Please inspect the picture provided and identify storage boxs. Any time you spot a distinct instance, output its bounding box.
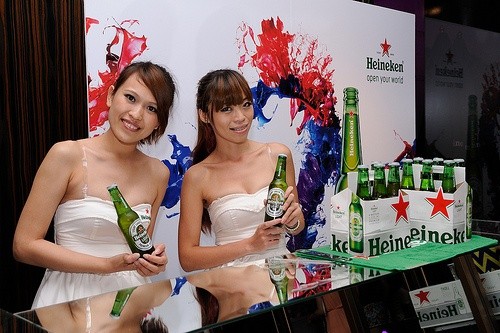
[330,166,473,258]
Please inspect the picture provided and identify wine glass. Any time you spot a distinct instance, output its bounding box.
[109,286,139,320]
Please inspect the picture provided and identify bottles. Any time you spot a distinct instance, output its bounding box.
[441,160,456,193]
[465,94,482,219]
[419,159,435,191]
[334,86,363,195]
[413,157,424,164]
[386,162,400,198]
[432,158,444,166]
[400,158,415,190]
[348,192,364,254]
[371,163,387,200]
[466,185,472,239]
[264,254,288,305]
[264,154,287,242]
[371,161,381,170]
[106,183,155,258]
[355,165,372,200]
[349,265,364,285]
[453,159,465,167]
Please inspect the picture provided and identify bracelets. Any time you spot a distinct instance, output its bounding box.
[283,220,300,232]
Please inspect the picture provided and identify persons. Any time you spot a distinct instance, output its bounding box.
[35,279,172,333]
[12,63,176,311]
[186,253,297,329]
[178,71,305,272]
